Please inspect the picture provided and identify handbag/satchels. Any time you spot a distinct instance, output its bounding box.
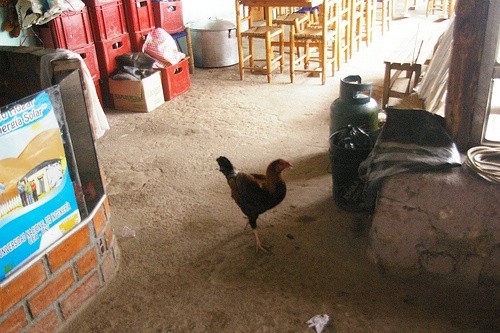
[142,27,186,69]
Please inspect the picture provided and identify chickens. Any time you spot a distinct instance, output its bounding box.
[215,155,294,255]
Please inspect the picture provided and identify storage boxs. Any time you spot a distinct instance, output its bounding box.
[35,0,191,112]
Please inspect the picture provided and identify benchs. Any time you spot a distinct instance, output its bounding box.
[381,35,431,110]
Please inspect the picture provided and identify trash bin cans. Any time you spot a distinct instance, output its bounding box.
[324,125,373,216]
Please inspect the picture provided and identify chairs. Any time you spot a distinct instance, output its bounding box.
[235,0,455,85]
